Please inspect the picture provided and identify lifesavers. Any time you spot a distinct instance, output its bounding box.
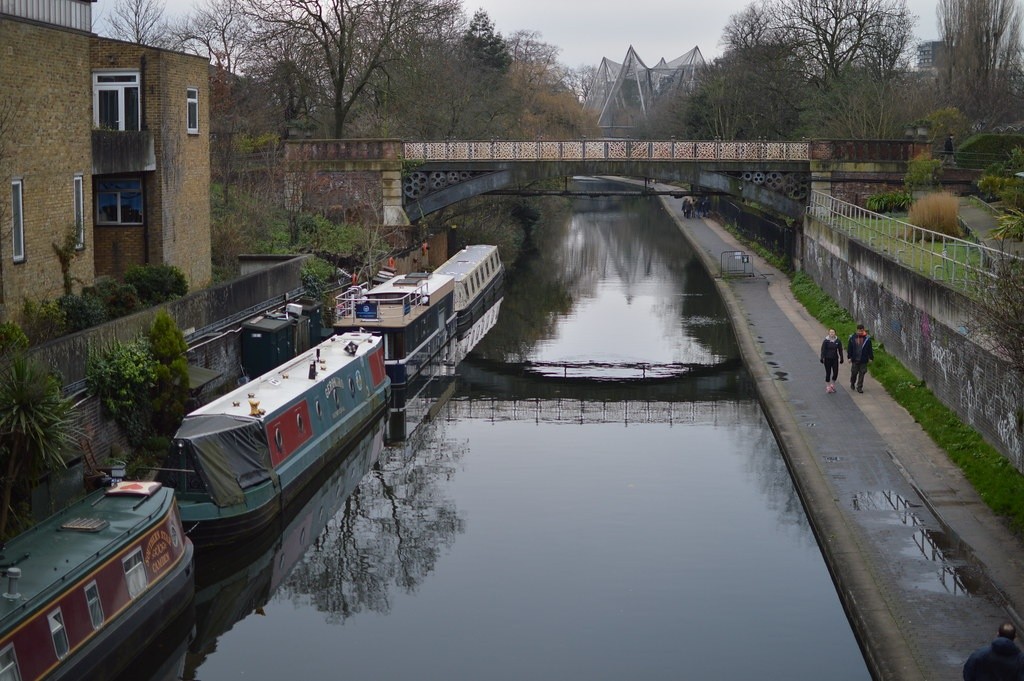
[422,242,428,254]
[352,272,358,286]
[388,256,395,270]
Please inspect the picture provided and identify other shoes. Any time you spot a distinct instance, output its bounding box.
[851,383,855,389]
[857,386,863,393]
[826,383,836,392]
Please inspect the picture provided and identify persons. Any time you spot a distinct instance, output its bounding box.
[681,197,710,219]
[847,325,874,393]
[941,134,954,167]
[820,328,843,393]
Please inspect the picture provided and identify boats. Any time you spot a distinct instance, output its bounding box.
[389,336,458,464]
[0,481,194,681]
[332,272,457,407]
[154,327,392,533]
[44,535,197,681]
[431,245,504,316]
[188,408,392,614]
[457,274,504,361]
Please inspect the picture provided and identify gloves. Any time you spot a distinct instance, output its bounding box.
[840,360,843,364]
[820,359,823,363]
